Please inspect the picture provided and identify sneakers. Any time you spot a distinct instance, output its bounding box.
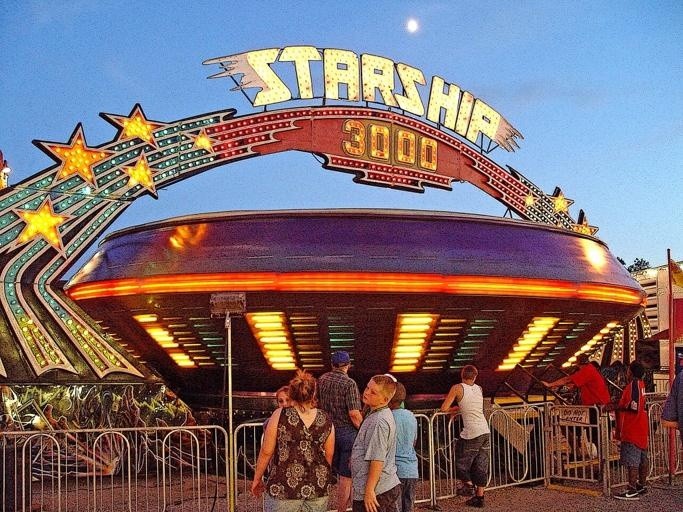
[614,482,649,500]
[456,483,485,507]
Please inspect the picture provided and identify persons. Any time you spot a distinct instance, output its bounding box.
[388,382,419,512]
[661,369,683,445]
[540,353,651,501]
[318,351,364,512]
[263,386,291,433]
[251,369,335,512]
[349,375,401,512]
[440,365,491,508]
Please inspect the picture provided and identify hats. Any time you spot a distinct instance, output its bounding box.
[571,353,588,364]
[331,351,350,363]
